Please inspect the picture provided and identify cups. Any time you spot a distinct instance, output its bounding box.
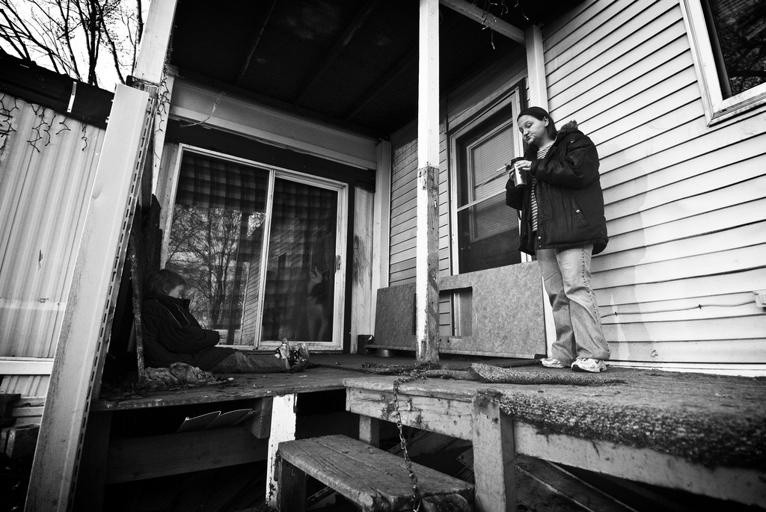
[510,156,529,188]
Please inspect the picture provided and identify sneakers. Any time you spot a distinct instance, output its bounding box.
[571,359,607,373]
[274,338,310,371]
[539,358,570,369]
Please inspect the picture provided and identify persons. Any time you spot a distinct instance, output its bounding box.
[142,269,311,373]
[505,107,612,372]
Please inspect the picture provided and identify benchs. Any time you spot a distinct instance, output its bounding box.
[271,432,475,512]
